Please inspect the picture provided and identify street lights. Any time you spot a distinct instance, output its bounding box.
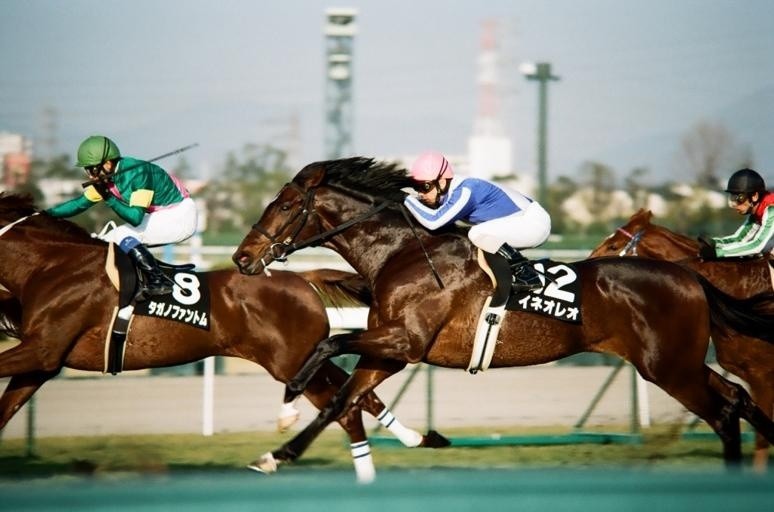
[319,3,359,159]
[526,57,560,235]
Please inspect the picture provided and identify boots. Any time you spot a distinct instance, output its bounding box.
[128,244,173,295]
[494,242,542,291]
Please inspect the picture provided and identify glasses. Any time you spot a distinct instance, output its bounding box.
[413,181,435,193]
[85,167,100,176]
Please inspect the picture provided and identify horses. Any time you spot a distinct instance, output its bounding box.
[0,191,452,484]
[230,156,774,476]
[588,208,774,473]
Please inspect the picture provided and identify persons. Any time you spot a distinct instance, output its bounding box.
[47,135,199,304]
[403,149,552,293]
[698,169,774,261]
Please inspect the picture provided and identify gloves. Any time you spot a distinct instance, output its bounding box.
[698,246,716,262]
[93,177,111,200]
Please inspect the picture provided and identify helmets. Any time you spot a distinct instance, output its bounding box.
[409,150,454,182]
[725,169,765,193]
[75,135,120,167]
